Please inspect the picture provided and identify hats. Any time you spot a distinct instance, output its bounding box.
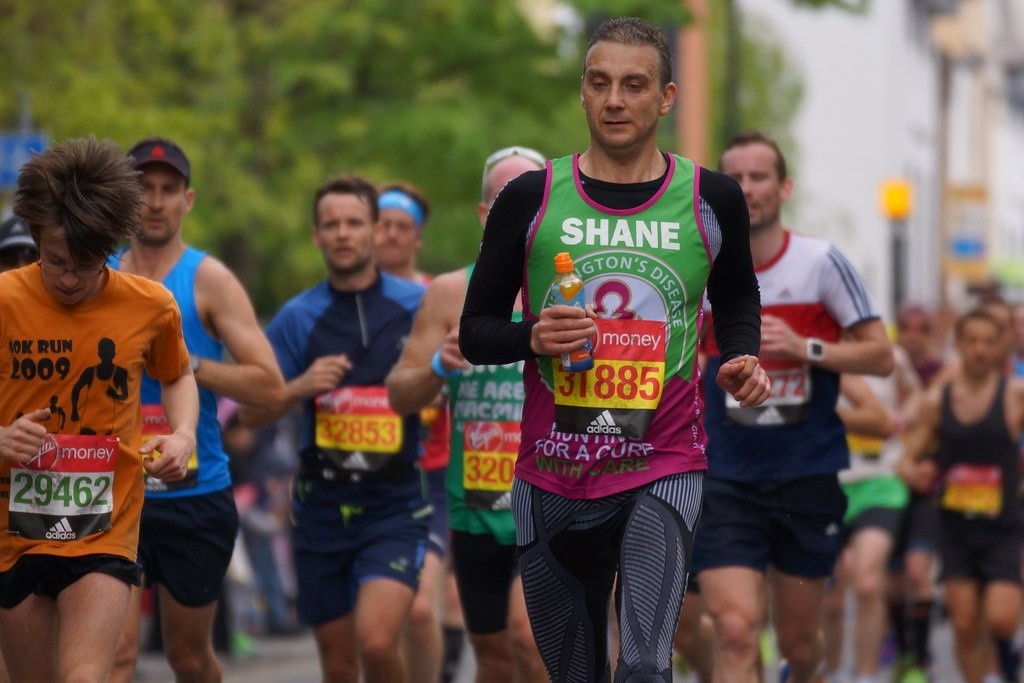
[125,137,191,186]
[0,217,37,249]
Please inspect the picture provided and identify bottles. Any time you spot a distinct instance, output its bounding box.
[549,252,594,372]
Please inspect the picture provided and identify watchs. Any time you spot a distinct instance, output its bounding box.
[189,355,200,372]
[803,338,825,370]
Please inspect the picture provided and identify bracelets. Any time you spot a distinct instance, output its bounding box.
[431,351,461,379]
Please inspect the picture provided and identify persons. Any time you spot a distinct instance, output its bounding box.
[0,139,285,683]
[218,146,559,683]
[461,15,771,683]
[677,133,1024,683]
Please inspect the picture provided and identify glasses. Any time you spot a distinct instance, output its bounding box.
[36,252,109,278]
